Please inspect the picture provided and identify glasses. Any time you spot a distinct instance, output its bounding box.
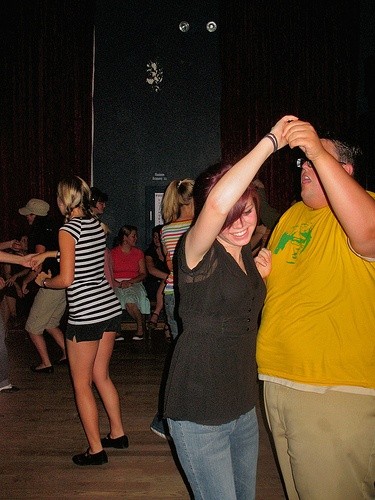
[296,157,347,169]
[154,234,160,239]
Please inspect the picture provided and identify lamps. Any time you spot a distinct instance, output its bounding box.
[145,56,164,95]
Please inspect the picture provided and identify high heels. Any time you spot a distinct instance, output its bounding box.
[148,312,159,327]
[162,327,173,345]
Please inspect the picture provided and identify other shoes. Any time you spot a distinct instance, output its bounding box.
[72,447,108,467]
[100,432,129,449]
[150,415,171,440]
[54,358,69,366]
[115,334,124,341]
[132,332,144,340]
[0,383,20,392]
[30,364,54,374]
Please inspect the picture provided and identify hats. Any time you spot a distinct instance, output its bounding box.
[18,199,50,216]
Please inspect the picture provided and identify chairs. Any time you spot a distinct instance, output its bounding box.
[119,302,168,341]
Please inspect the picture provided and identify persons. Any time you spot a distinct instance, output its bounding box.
[250,178,303,259]
[0,173,197,466]
[255,121,375,500]
[161,114,299,500]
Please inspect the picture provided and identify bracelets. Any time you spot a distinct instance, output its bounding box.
[263,133,279,154]
[258,183,264,188]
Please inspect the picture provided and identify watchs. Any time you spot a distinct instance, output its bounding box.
[43,278,51,289]
[119,282,122,288]
